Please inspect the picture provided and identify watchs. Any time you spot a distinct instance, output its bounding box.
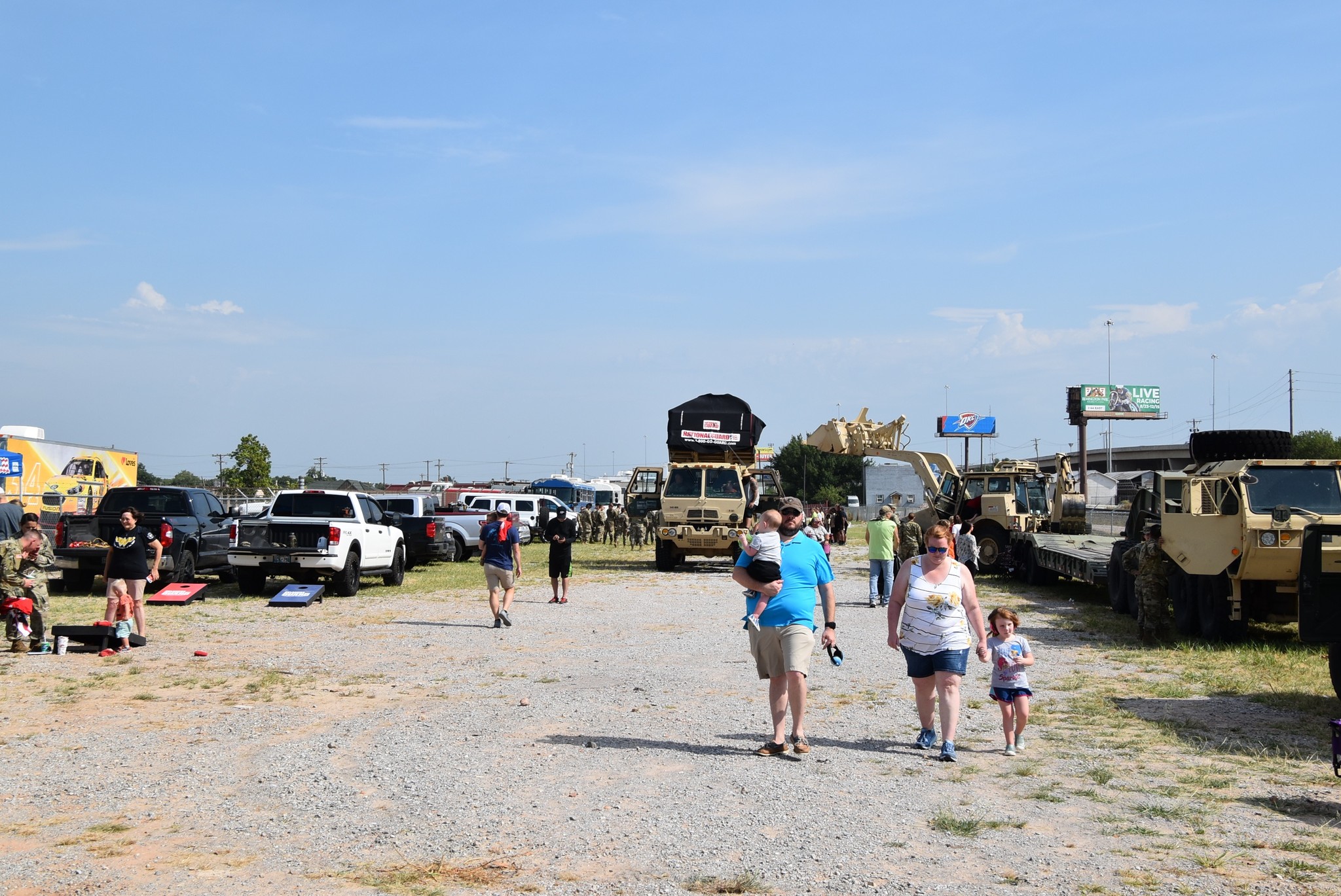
[825,621,836,629]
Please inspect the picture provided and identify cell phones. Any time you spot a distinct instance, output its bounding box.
[558,538,563,540]
[28,547,40,556]
[977,546,981,549]
[146,574,153,583]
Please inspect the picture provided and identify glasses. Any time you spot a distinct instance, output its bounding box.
[119,518,131,522]
[782,508,800,516]
[928,546,948,554]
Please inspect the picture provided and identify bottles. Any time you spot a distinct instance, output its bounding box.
[288,533,296,548]
[317,536,327,550]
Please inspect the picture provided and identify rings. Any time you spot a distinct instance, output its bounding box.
[834,644,835,645]
[31,555,33,557]
[751,506,752,506]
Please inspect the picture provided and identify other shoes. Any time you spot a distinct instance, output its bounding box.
[479,558,484,566]
[115,645,132,653]
[583,539,654,551]
[1006,744,1016,756]
[1014,730,1026,750]
[742,589,756,599]
[869,601,888,607]
[748,614,760,632]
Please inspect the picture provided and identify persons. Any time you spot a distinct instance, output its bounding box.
[576,502,660,550]
[103,506,163,637]
[886,503,901,556]
[479,502,522,628]
[0,529,43,653]
[732,496,836,756]
[937,515,980,580]
[111,579,133,653]
[721,468,760,529]
[545,505,576,604]
[724,480,739,492]
[978,608,1034,756]
[886,525,988,762]
[800,503,849,546]
[5,513,56,649]
[865,505,900,608]
[0,486,27,542]
[479,513,498,566]
[899,512,923,564]
[739,510,783,633]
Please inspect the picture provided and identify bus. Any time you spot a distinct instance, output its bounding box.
[523,474,597,515]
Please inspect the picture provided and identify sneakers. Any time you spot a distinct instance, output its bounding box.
[939,740,958,762]
[790,733,810,754]
[499,610,511,626]
[756,741,788,756]
[548,597,559,603]
[494,620,501,628]
[30,639,52,651]
[10,640,30,652]
[915,727,936,750]
[559,598,568,604]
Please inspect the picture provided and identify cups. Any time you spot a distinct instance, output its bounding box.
[57,636,69,655]
[41,642,50,652]
[738,534,753,550]
[25,575,36,579]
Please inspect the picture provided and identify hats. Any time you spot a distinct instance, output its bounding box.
[741,469,752,477]
[887,503,897,508]
[778,497,803,512]
[497,502,511,514]
[879,506,893,514]
[557,507,566,519]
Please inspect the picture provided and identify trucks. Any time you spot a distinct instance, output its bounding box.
[623,393,790,569]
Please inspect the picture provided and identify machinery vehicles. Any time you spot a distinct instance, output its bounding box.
[801,408,1056,577]
[1018,427,1341,647]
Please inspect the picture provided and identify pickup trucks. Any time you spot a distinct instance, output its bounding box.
[366,489,582,570]
[228,488,407,597]
[50,484,240,592]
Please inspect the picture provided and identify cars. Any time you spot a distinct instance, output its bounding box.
[40,456,110,518]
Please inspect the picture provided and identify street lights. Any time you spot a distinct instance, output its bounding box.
[583,442,587,481]
[944,385,950,456]
[1211,354,1219,430]
[612,450,616,476]
[1104,319,1114,473]
[643,435,648,467]
